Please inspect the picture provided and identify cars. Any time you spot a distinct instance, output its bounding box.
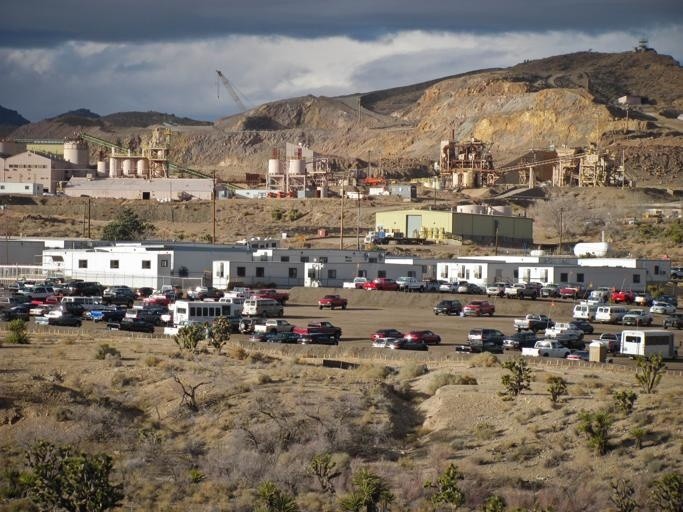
[435,300,462,316]
[370,328,441,351]
[1,277,178,333]
[590,286,653,305]
[661,314,683,328]
[503,332,538,350]
[298,334,338,345]
[671,268,683,279]
[601,332,620,350]
[650,303,677,314]
[427,280,454,291]
[546,319,593,350]
[652,294,677,305]
[458,281,470,292]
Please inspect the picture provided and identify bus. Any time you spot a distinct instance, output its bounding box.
[173,301,244,328]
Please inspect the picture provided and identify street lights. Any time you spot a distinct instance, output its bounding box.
[81,194,91,238]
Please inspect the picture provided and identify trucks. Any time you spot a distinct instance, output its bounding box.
[573,304,598,321]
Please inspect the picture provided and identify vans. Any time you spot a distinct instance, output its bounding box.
[243,299,284,317]
[596,306,628,324]
[468,329,510,346]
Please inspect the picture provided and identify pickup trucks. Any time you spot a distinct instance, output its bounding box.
[342,276,367,289]
[623,309,653,327]
[464,300,495,317]
[396,276,427,292]
[522,339,571,358]
[307,321,341,336]
[487,282,585,300]
[250,288,289,301]
[514,314,555,331]
[255,320,294,334]
[319,295,347,310]
[362,278,397,289]
